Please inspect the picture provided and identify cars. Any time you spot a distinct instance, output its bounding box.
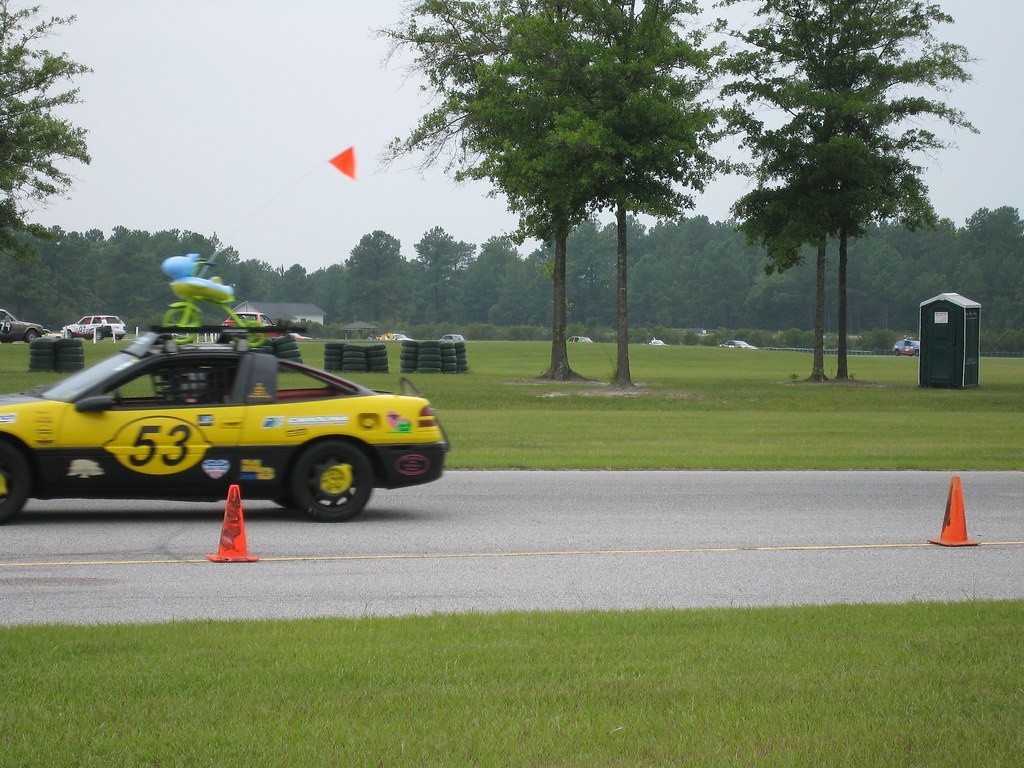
[718,340,758,349]
[0,309,45,344]
[566,336,593,343]
[61,315,128,340]
[649,338,665,345]
[892,339,919,357]
[439,334,465,341]
[0,325,450,523]
[42,329,62,340]
[223,312,415,341]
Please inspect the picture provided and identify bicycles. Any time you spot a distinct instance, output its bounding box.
[161,253,266,348]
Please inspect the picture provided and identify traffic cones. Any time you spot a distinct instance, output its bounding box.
[927,477,981,547]
[207,485,259,563]
